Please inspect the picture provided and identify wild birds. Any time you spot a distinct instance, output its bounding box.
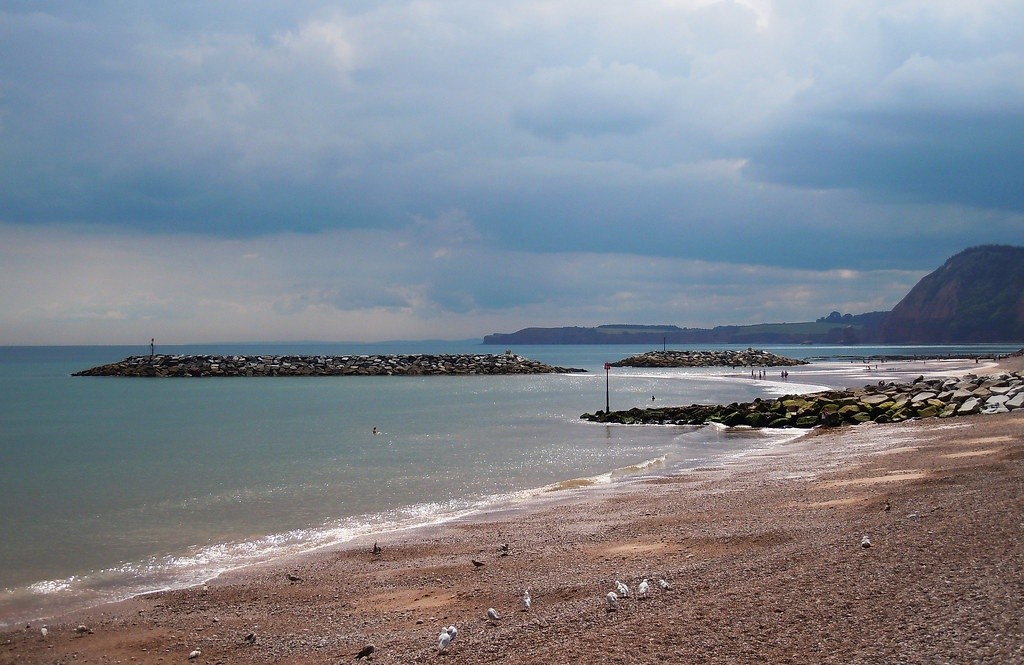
[614,580,629,599]
[189,647,201,659]
[40,625,48,639]
[500,544,510,555]
[245,630,257,645]
[437,625,458,654]
[657,580,673,591]
[471,560,485,571]
[638,579,650,599]
[860,535,871,549]
[487,608,499,625]
[606,592,618,612]
[884,503,891,512]
[202,583,209,595]
[73,625,90,637]
[353,644,375,662]
[373,543,381,554]
[522,592,531,612]
[286,574,302,586]
